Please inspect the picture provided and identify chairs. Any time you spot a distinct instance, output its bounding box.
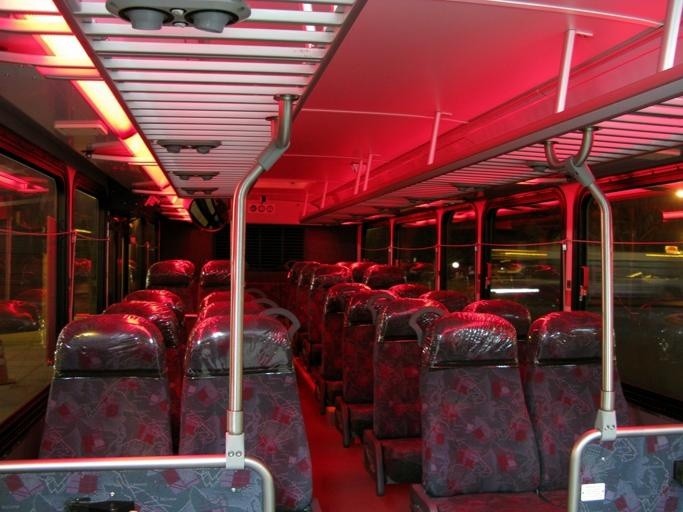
[0,249,682,512]
